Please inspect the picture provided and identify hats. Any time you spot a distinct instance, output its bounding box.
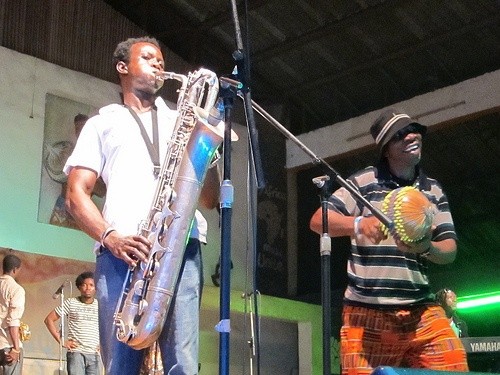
[370,109,428,161]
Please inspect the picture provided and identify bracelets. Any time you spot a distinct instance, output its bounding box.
[353,216,365,240]
[100,227,116,249]
[11,348,20,354]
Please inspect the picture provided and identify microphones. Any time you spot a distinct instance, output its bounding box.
[52,280,67,299]
[241,289,258,297]
[218,76,243,89]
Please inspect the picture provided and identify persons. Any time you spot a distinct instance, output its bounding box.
[0,255,30,375]
[44,271,105,375]
[307,109,471,373]
[64,37,222,374]
[50,112,106,229]
[434,287,469,339]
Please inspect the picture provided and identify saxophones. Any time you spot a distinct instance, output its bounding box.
[111,66,240,351]
